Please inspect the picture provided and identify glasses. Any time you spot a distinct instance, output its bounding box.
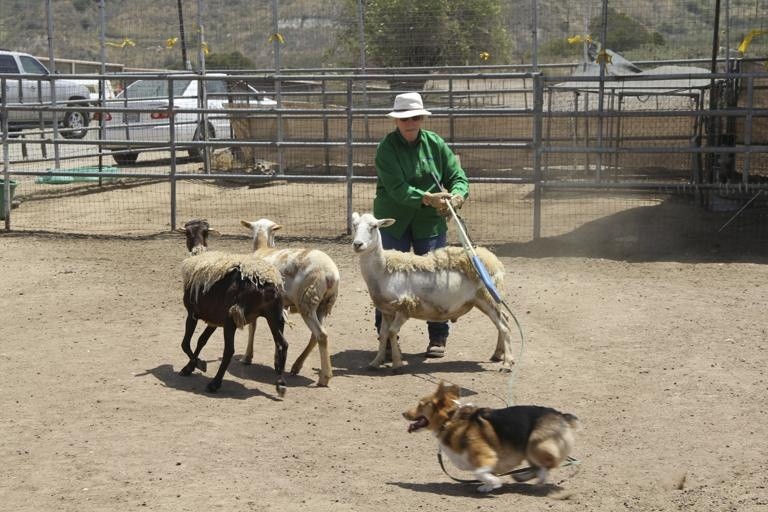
[399,116,421,121]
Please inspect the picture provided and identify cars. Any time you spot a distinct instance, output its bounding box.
[72,73,115,120]
[0,49,90,140]
[102,72,277,166]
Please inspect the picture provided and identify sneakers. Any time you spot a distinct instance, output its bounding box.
[379,336,402,361]
[426,337,445,357]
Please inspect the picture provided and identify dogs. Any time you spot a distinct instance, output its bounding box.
[401,381,584,493]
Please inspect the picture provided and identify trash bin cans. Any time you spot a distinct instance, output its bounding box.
[0,179,18,220]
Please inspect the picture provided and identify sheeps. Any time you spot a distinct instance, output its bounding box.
[238,217,340,387]
[175,218,290,398]
[351,211,517,374]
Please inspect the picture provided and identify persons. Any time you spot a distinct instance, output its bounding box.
[368,91,470,359]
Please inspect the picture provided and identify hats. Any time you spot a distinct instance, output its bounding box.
[384,92,432,118]
